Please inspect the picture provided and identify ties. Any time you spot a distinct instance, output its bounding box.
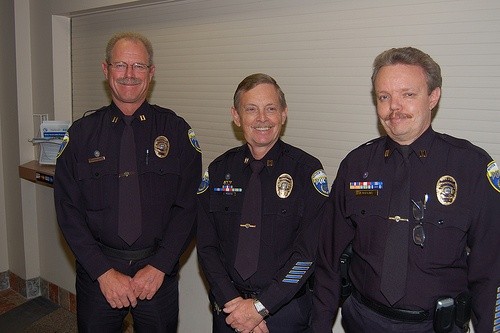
[234,160,264,281]
[380,145,413,305]
[118,116,142,246]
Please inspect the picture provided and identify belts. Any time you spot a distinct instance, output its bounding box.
[95,242,160,259]
[239,282,309,299]
[351,285,435,322]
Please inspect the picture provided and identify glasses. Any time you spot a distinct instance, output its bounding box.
[411,200,425,247]
[107,62,152,72]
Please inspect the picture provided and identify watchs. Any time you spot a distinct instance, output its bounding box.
[253,297,268,318]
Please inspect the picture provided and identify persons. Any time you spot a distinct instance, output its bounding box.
[330,47,500,333]
[53,32,202,333]
[194,74,339,333]
